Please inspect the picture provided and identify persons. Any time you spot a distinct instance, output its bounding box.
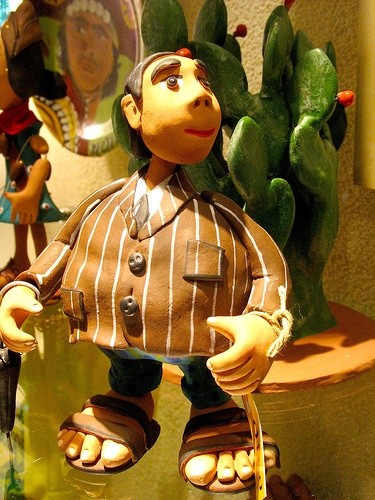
[0,49,294,493]
